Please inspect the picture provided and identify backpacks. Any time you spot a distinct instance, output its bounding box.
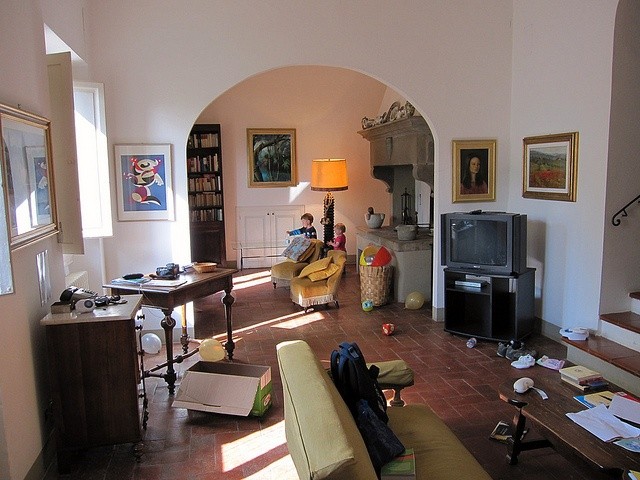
[331,342,389,425]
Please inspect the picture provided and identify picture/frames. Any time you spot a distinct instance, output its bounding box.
[113,143,175,222]
[523,132,579,202]
[0,153,15,295]
[453,140,497,201]
[247,128,297,186]
[0,103,59,252]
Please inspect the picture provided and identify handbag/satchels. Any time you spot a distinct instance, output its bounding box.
[356,399,405,471]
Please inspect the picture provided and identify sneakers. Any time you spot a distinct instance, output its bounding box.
[505,342,539,362]
[497,339,519,357]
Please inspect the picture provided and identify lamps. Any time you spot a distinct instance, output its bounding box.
[311,159,348,247]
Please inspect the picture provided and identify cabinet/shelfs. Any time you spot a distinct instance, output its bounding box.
[443,268,536,344]
[186,124,227,267]
[40,295,148,463]
[236,206,305,268]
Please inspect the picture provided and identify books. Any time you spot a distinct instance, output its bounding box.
[187,153,220,172]
[381,447,415,480]
[193,208,223,221]
[559,365,603,390]
[584,391,618,407]
[573,395,595,409]
[187,133,218,148]
[191,192,223,206]
[189,173,222,191]
[490,419,529,445]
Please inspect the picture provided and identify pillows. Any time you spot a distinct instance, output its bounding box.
[298,255,332,278]
[282,236,310,261]
[298,241,316,261]
[285,248,315,263]
[308,263,339,282]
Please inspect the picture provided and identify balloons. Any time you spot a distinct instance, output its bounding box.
[141,333,163,353]
[199,338,227,362]
[404,292,423,311]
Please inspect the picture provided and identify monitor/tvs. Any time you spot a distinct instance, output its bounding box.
[440,212,528,275]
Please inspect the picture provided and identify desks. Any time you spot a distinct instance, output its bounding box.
[497,373,640,480]
[356,225,433,303]
[103,267,238,394]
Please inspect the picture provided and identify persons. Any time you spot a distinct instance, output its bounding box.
[287,213,317,240]
[461,154,489,193]
[327,223,347,274]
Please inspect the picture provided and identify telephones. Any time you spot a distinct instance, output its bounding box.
[57,286,98,307]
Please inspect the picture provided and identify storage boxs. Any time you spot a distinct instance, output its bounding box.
[172,361,273,419]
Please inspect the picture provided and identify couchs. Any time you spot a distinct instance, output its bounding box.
[270,240,322,289]
[275,340,492,480]
[291,250,346,313]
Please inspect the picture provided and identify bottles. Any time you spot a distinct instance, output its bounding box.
[284,232,290,248]
